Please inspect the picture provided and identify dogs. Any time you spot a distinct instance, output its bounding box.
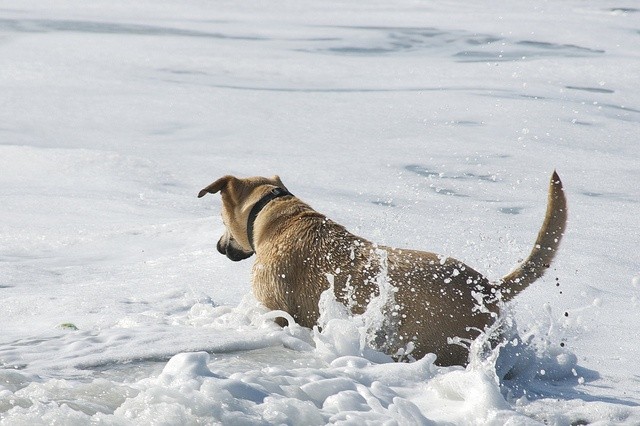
[198,168,567,367]
